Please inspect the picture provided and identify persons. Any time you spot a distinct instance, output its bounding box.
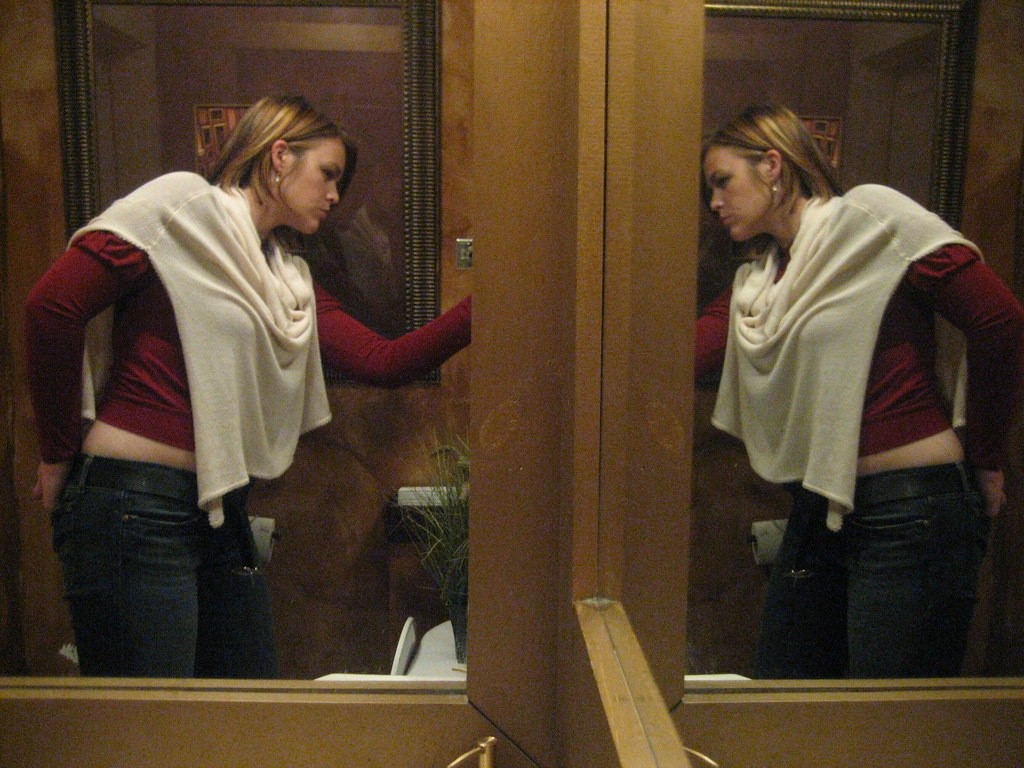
[695,99,1024,677]
[19,91,473,677]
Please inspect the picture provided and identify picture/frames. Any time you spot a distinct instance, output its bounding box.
[52,0,444,389]
[717,0,979,315]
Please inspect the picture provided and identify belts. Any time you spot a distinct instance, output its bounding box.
[72,460,197,504]
[855,467,974,508]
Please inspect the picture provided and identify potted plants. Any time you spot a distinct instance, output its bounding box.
[386,418,469,664]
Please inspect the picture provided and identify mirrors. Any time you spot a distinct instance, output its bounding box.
[0,0,657,768]
[603,0,1024,768]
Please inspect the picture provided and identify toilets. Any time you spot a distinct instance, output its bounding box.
[391,617,466,679]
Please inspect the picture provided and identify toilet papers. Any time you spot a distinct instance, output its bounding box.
[248,516,275,564]
[752,519,788,565]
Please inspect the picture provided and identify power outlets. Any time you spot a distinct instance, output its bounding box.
[456,239,473,269]
